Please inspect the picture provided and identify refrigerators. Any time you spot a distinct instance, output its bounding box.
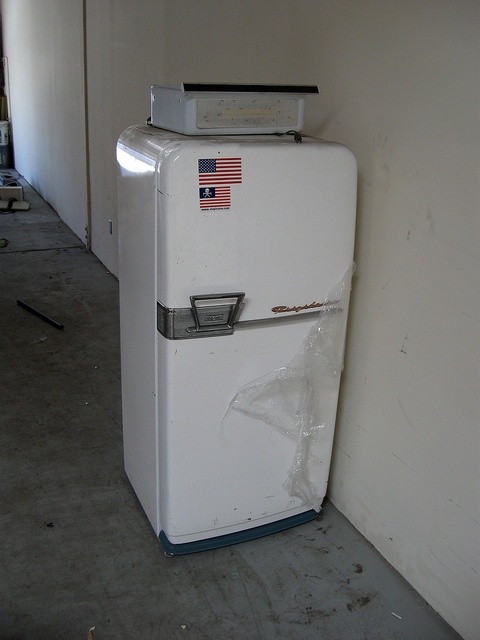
[116,126,360,557]
